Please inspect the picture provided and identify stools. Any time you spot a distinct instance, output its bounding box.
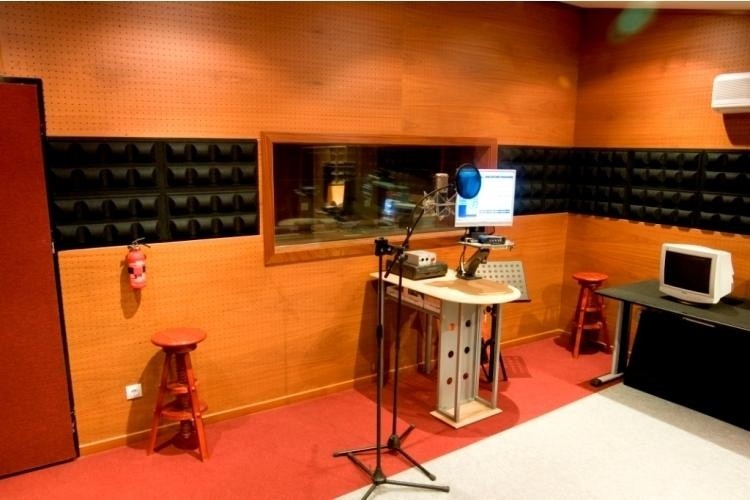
[572,272,613,359]
[147,327,209,461]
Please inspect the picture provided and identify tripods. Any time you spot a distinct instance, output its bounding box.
[332,245,436,482]
[347,239,449,500]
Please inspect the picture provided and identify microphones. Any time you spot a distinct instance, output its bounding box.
[433,172,448,217]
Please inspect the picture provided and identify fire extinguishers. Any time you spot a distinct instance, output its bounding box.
[126,238,150,289]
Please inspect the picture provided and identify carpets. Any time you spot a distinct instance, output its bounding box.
[0,335,626,500]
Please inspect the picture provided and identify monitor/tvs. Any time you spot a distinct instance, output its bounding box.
[658,243,734,305]
[454,168,517,242]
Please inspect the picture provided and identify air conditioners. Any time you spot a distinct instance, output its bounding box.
[707,72,750,112]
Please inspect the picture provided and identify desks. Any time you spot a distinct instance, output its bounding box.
[588,280,750,398]
[369,272,522,429]
